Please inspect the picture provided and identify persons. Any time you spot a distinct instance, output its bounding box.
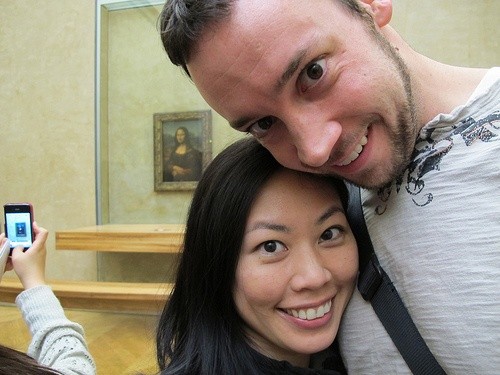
[156,0,500,375]
[155,136,359,375]
[0,221,96,375]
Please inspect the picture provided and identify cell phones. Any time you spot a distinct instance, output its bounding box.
[3,204,35,255]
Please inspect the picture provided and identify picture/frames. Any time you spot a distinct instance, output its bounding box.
[153,109,213,191]
